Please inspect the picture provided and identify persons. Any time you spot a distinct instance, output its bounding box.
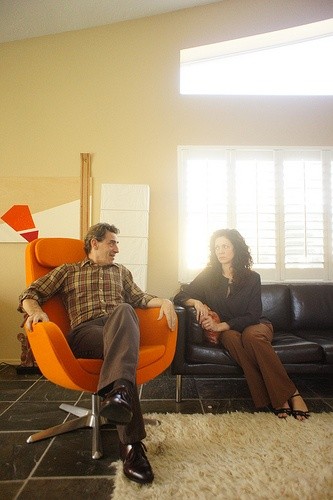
[173,229,311,421]
[17,222,176,486]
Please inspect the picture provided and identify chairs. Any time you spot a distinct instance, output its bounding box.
[25,238,178,459]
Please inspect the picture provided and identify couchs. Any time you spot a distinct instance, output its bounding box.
[173,281,333,403]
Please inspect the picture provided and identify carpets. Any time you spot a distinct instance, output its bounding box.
[111,411,333,499]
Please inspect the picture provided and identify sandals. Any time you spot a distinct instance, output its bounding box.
[290,393,310,420]
[273,403,291,418]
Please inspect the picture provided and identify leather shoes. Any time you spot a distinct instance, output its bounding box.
[118,439,155,483]
[100,385,134,422]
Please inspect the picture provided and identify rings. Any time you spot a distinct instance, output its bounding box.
[200,311,205,316]
